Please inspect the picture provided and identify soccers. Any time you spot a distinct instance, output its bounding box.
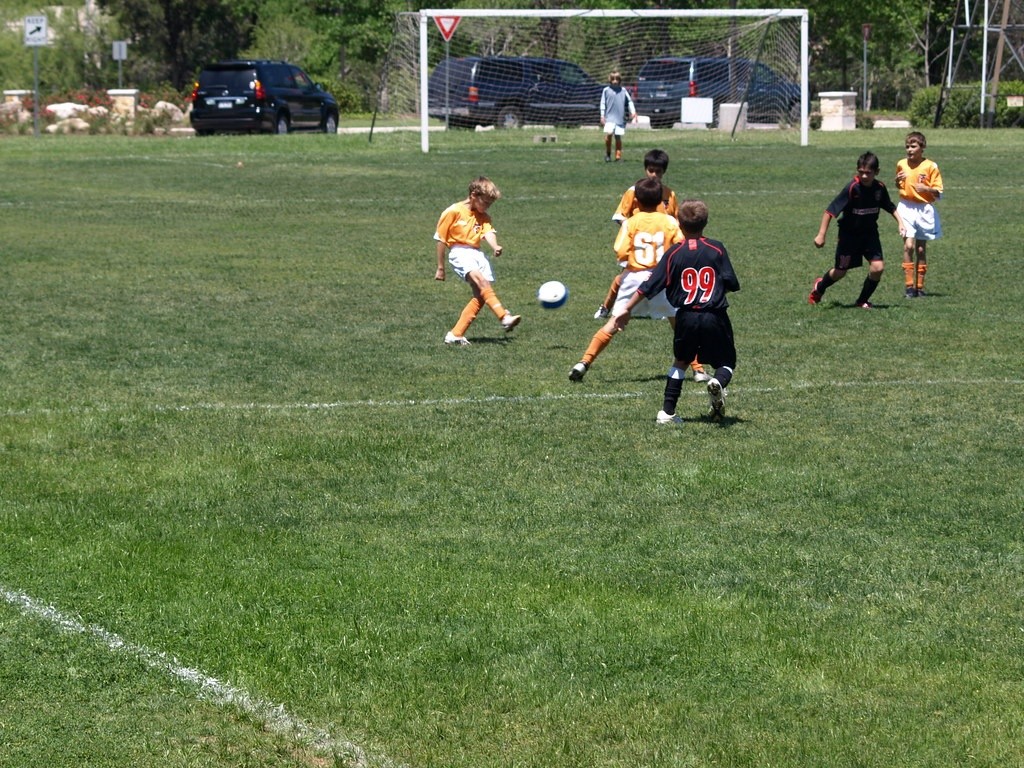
[538,280,567,309]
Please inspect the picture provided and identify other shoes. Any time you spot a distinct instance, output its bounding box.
[605,154,611,162]
[616,157,621,163]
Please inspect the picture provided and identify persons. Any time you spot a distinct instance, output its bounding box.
[433,177,521,345]
[568,178,711,382]
[895,132,943,297]
[594,149,679,320]
[809,151,907,309]
[600,72,638,163]
[614,199,740,424]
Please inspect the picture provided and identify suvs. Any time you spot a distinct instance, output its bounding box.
[429,54,632,132]
[190,59,339,135]
[630,55,814,130]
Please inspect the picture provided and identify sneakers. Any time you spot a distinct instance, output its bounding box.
[855,299,872,310]
[568,363,587,381]
[445,333,471,347]
[904,288,916,298]
[693,371,712,382]
[917,289,926,297]
[501,311,523,333]
[707,377,725,418]
[656,409,683,423]
[594,305,608,319]
[809,277,825,304]
